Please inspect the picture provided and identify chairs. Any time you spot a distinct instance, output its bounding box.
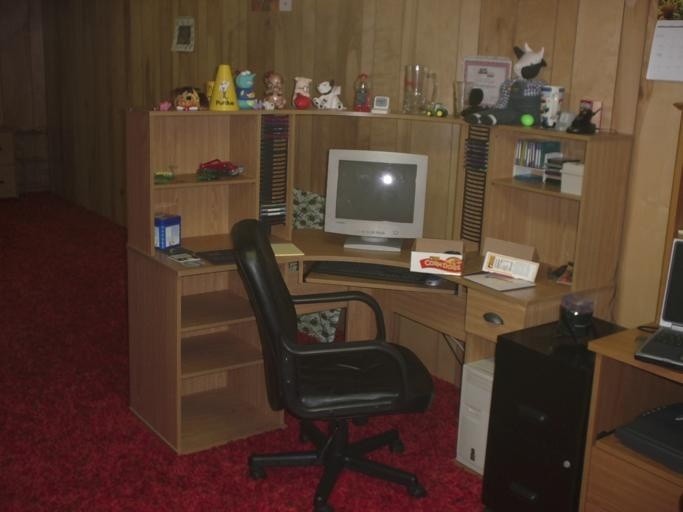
[230,220,435,511]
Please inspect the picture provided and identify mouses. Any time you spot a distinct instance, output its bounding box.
[425,275,443,287]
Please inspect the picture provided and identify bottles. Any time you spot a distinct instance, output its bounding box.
[354,73,372,112]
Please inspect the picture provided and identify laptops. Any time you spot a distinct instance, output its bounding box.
[634,238,683,372]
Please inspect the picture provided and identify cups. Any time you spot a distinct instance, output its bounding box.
[558,295,595,326]
[453,80,475,118]
[538,84,564,128]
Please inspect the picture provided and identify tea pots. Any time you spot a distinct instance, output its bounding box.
[402,65,438,115]
[204,61,239,111]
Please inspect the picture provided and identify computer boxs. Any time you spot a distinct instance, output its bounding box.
[457,357,496,475]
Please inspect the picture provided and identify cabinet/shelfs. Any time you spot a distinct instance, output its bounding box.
[450,112,635,484]
[481,315,628,511]
[123,104,294,455]
[575,99,682,512]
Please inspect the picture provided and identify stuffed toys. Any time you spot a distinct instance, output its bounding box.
[463,42,547,127]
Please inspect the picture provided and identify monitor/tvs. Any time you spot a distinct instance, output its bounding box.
[325,149,427,252]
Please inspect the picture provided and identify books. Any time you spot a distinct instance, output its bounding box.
[269,242,305,256]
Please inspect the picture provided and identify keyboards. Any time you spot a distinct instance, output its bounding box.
[311,261,417,283]
